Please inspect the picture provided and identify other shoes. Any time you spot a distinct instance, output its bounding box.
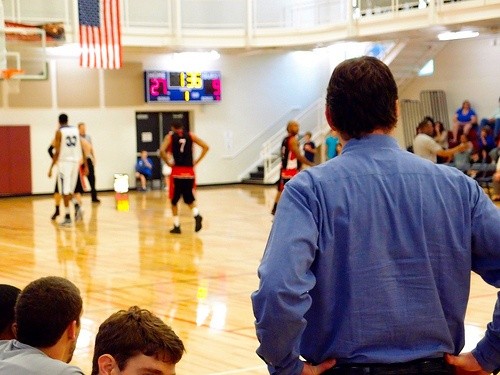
[61,219,72,224]
[52,212,59,220]
[75,207,82,221]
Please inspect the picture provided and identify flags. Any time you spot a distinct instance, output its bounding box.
[77,0,123,70]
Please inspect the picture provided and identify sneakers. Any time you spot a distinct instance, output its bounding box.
[170,226,181,233]
[194,215,202,231]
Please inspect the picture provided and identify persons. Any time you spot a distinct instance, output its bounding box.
[250,55,500,375]
[160,117,209,234]
[135,129,176,191]
[48,114,101,222]
[271,100,500,224]
[0,276,186,375]
